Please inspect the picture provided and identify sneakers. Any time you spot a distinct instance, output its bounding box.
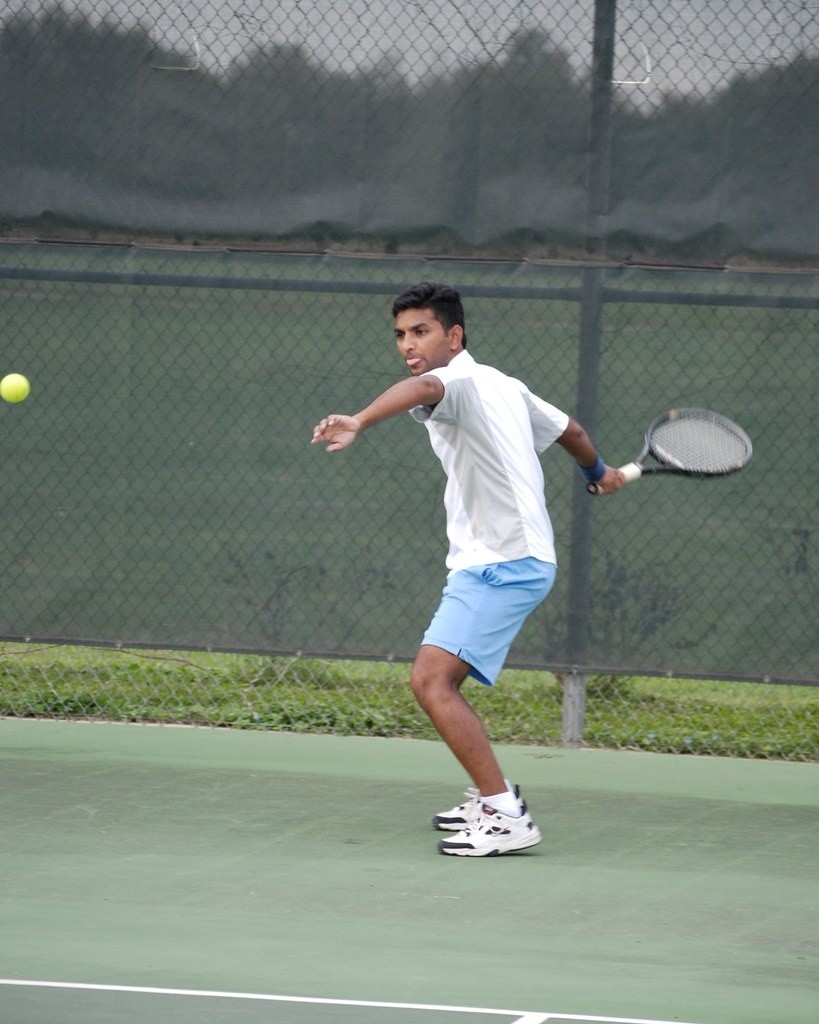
[438,802,542,857]
[433,785,519,831]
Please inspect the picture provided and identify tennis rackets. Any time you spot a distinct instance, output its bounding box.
[584,406,755,496]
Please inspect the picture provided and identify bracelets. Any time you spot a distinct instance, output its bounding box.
[577,453,605,481]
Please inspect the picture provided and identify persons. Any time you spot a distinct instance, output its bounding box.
[310,282,627,859]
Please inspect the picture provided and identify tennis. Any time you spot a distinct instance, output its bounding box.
[1,372,29,403]
